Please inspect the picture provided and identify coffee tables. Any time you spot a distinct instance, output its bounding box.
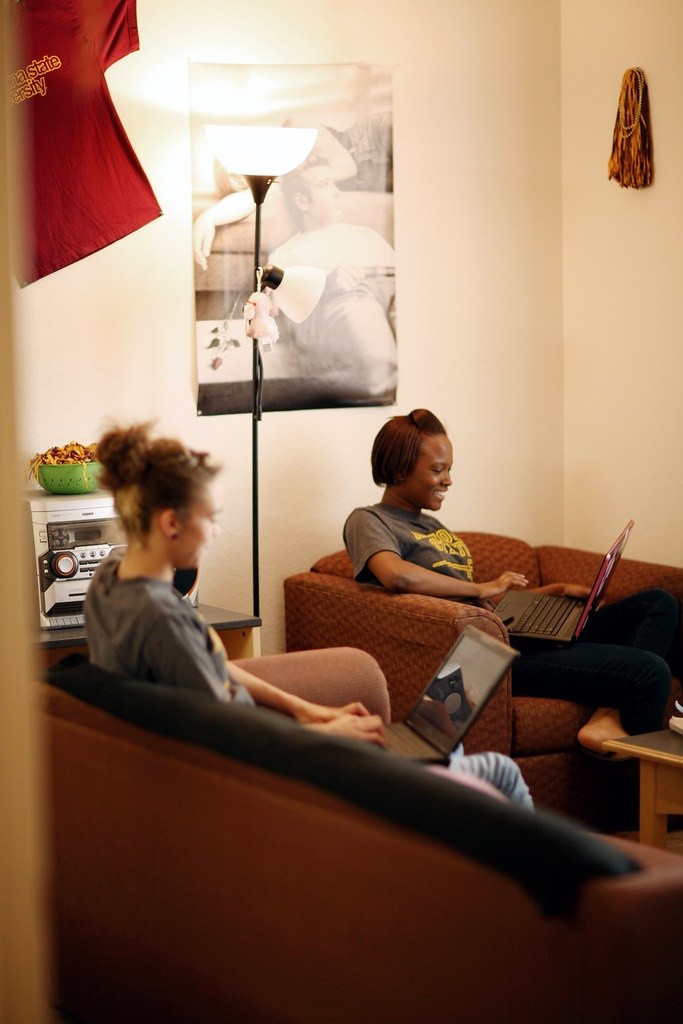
[602,731,683,856]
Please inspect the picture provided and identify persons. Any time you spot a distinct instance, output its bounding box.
[270,154,395,372]
[83,426,535,811]
[193,112,393,272]
[342,408,672,760]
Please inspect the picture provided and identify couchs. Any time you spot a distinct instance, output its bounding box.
[282,532,683,832]
[40,651,683,1024]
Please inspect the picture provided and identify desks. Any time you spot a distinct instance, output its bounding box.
[42,602,262,685]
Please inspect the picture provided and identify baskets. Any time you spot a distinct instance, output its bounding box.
[33,462,101,494]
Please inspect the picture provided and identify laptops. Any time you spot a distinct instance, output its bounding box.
[492,520,634,644]
[381,625,520,766]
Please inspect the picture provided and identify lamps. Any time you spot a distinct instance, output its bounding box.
[209,122,326,658]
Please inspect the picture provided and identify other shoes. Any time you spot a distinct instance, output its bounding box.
[571,736,640,796]
